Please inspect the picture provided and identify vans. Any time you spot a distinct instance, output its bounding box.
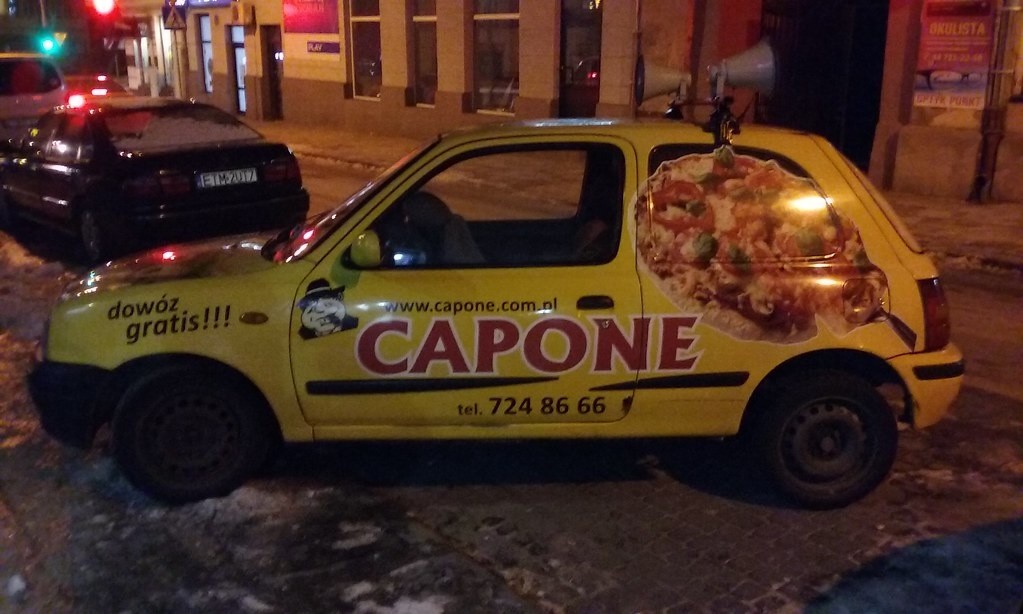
[0,53,70,150]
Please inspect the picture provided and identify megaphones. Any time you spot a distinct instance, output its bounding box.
[706,38,777,134]
[634,55,692,121]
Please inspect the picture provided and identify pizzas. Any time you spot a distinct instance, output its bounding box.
[628,144,890,344]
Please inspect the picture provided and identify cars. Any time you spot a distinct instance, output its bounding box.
[23,118,967,508]
[0,94,310,272]
[68,77,136,118]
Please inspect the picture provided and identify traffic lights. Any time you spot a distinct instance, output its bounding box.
[38,34,58,54]
[88,0,122,19]
